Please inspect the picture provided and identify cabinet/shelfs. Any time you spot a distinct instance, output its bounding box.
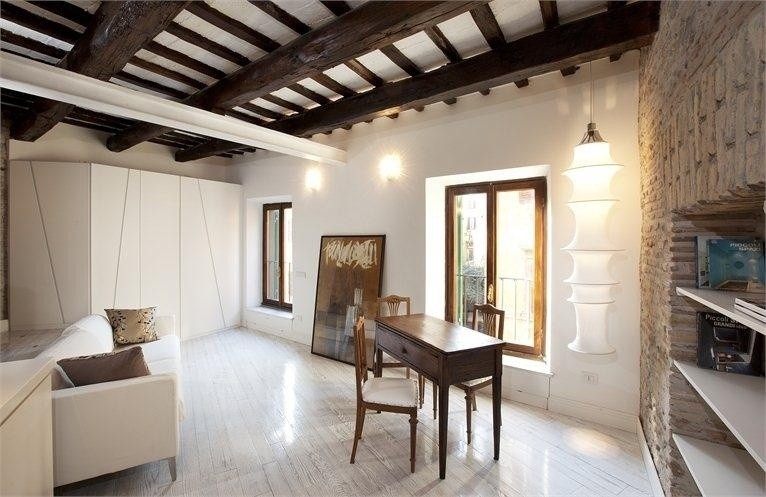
[0,357,56,497]
[671,286,766,497]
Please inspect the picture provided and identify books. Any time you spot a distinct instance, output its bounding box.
[734,296,766,316]
[706,238,766,293]
[693,234,762,290]
[734,303,766,323]
[695,310,762,378]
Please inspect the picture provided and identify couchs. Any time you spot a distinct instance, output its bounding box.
[35,314,181,488]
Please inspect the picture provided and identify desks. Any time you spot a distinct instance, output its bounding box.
[374,313,507,480]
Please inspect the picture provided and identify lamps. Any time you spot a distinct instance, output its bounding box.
[561,61,625,355]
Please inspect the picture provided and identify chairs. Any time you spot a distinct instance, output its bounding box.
[433,303,505,444]
[349,318,418,473]
[373,296,423,409]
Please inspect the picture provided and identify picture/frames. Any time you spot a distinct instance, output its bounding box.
[310,234,386,371]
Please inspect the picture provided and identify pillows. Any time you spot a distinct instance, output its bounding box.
[56,346,151,388]
[104,306,161,346]
